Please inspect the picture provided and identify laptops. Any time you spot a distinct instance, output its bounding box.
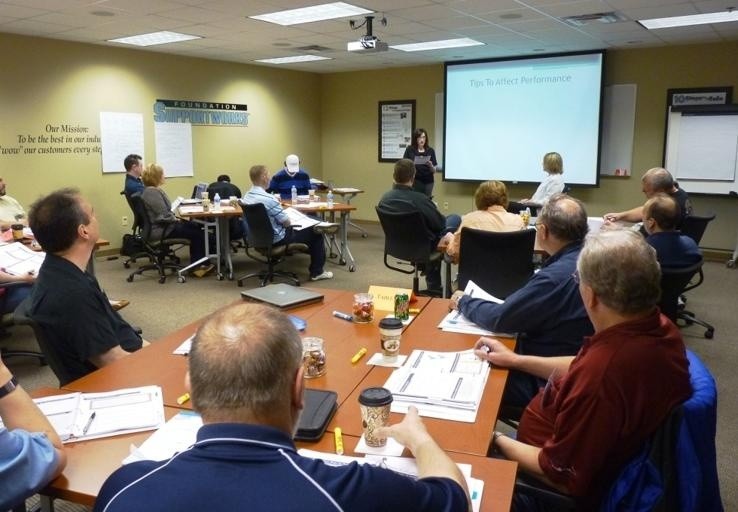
[180,183,207,204]
[240,282,324,311]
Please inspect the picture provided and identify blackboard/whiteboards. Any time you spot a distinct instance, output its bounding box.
[434,83,637,178]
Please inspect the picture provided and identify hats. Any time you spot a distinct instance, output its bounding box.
[286,154,299,173]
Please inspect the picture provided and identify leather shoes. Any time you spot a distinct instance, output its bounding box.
[194,264,215,278]
[111,299,129,311]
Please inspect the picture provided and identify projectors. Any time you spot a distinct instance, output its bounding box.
[347,36,388,53]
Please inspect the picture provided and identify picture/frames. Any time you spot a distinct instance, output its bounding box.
[377,98,417,164]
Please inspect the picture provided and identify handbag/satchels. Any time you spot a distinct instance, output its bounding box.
[119,232,149,257]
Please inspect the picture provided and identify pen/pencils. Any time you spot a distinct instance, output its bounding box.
[83,413,95,432]
[333,310,353,321]
[177,393,190,405]
[334,427,344,455]
[607,216,617,219]
[410,308,420,316]
[351,348,368,363]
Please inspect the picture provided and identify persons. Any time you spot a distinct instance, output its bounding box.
[0,354,68,512]
[378,159,463,295]
[265,154,311,199]
[602,168,699,295]
[509,152,565,216]
[0,241,129,317]
[135,164,210,268]
[206,174,241,200]
[445,180,525,266]
[29,188,151,389]
[0,177,29,234]
[403,129,436,199]
[123,154,144,228]
[240,164,333,281]
[91,300,473,512]
[473,226,692,512]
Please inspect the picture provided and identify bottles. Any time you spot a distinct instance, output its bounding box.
[352,293,375,325]
[301,337,327,380]
[229,195,238,208]
[214,193,221,209]
[291,185,298,203]
[327,190,334,208]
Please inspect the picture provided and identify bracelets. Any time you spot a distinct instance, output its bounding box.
[0,377,18,401]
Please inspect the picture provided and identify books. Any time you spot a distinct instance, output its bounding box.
[0,242,47,279]
[0,385,167,445]
[381,345,494,422]
[279,206,320,233]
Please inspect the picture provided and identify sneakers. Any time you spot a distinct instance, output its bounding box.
[311,270,334,281]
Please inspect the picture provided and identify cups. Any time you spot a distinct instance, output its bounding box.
[11,224,24,243]
[201,191,210,211]
[308,190,315,200]
[358,386,393,448]
[378,318,403,363]
